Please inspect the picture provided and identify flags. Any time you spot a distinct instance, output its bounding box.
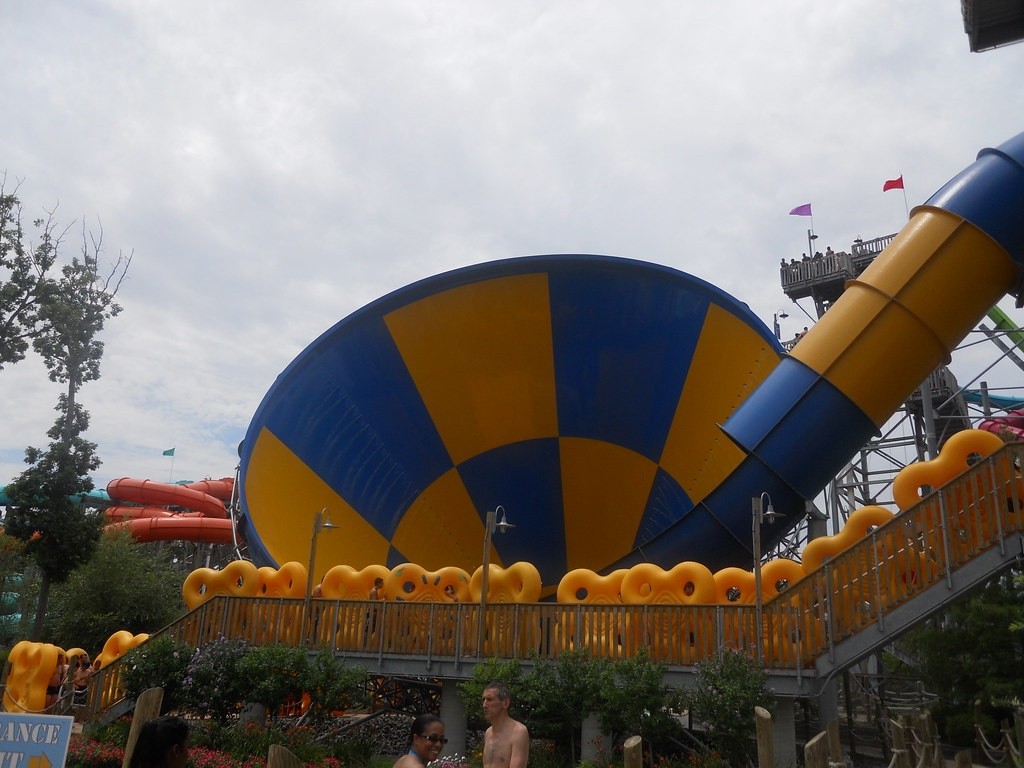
[789,204,811,216]
[883,177,904,192]
[163,448,174,456]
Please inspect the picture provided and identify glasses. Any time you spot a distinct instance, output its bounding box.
[418,735,448,744]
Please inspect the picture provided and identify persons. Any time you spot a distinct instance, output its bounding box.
[802,253,810,261]
[812,252,823,262]
[792,327,808,348]
[826,247,834,256]
[790,259,801,266]
[482,682,529,768]
[46,653,100,708]
[393,714,445,768]
[780,258,788,267]
